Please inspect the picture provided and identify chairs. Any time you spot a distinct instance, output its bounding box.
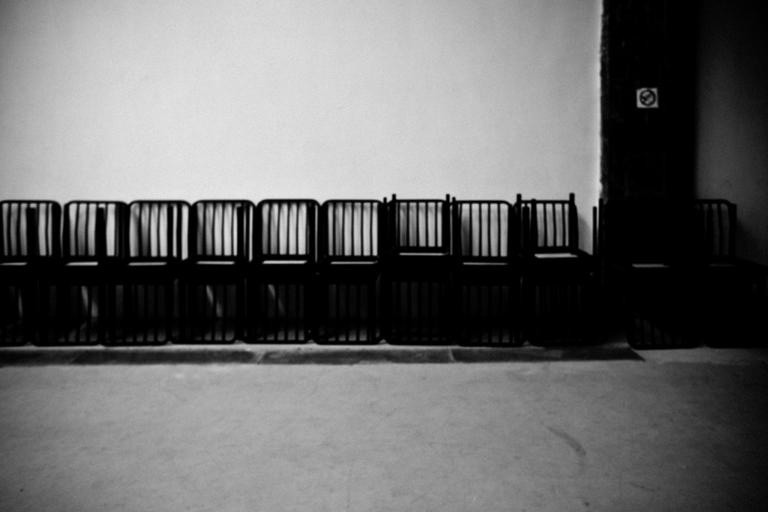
[1,192,745,360]
